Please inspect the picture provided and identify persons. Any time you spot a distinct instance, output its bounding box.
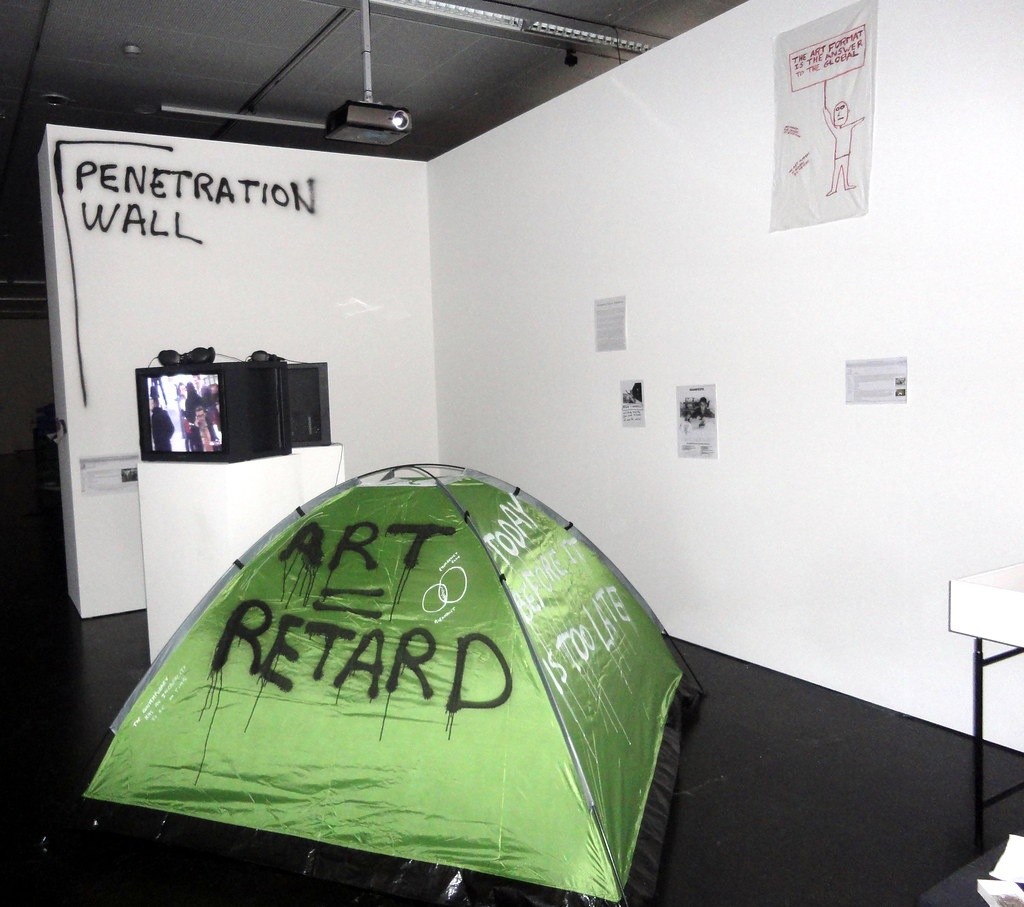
[150,382,219,451]
[689,397,715,421]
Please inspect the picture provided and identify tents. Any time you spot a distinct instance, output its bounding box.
[30,465,706,907]
